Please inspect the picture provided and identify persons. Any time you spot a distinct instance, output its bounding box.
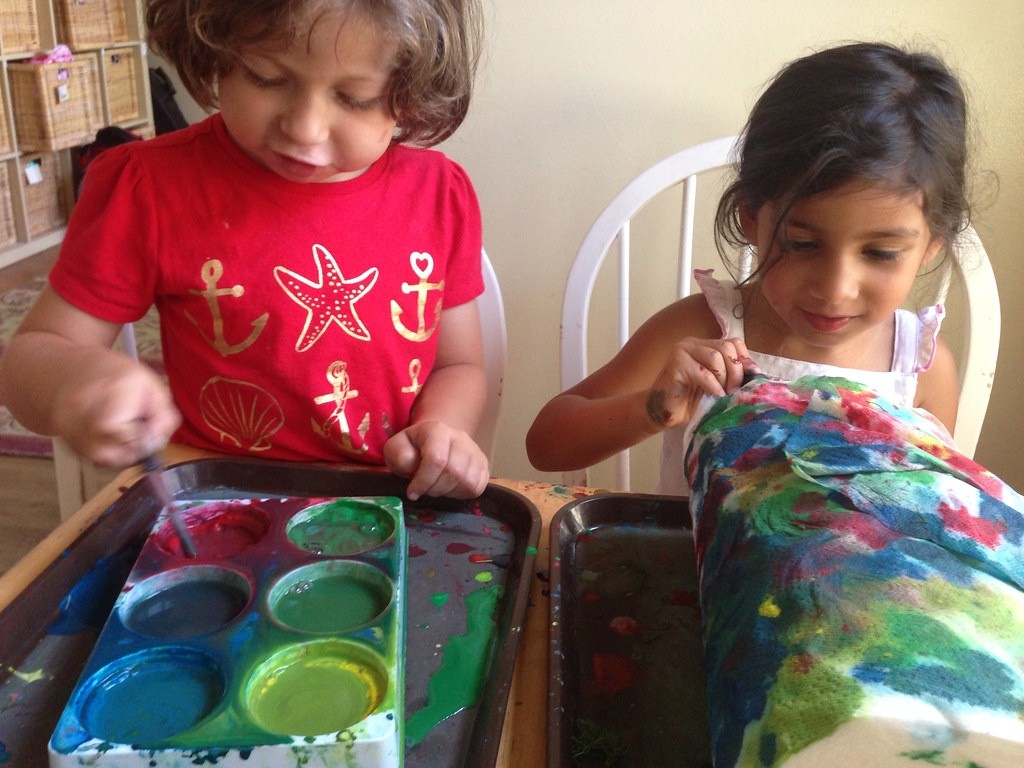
[0,0,492,503]
[523,36,997,473]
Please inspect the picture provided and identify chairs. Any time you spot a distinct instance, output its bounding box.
[48,245,509,532]
[560,133,1003,498]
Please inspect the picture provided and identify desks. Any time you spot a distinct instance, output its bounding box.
[1,440,653,768]
